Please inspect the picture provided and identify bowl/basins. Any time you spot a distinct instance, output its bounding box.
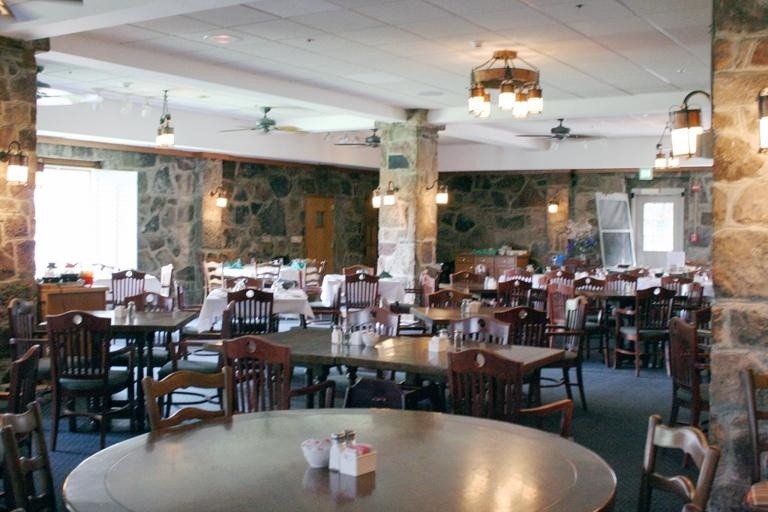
[363,333,378,348]
[302,439,330,469]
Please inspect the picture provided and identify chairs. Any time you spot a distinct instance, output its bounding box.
[634,415,722,510]
[0,399,57,510]
[221,336,336,411]
[141,364,233,428]
[742,369,766,510]
[110,248,712,377]
[154,300,237,420]
[448,349,575,439]
[1,344,41,456]
[344,377,408,408]
[46,311,136,450]
[8,299,89,430]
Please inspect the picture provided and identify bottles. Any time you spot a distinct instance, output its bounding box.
[453,329,462,351]
[330,325,342,346]
[42,262,61,283]
[329,429,361,473]
[342,326,349,347]
[460,299,471,313]
[60,263,80,282]
[127,302,135,318]
[438,329,448,337]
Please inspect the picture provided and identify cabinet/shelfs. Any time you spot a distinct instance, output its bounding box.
[39,284,109,317]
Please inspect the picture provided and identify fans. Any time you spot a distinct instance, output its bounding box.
[333,128,380,147]
[221,106,309,135]
[516,119,604,150]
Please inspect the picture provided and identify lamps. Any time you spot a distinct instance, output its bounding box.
[655,121,678,168]
[373,186,381,208]
[757,87,768,153]
[467,50,546,118]
[209,185,227,207]
[155,88,178,146]
[427,179,448,205]
[548,195,559,213]
[384,180,399,205]
[2,141,30,185]
[669,90,711,159]
